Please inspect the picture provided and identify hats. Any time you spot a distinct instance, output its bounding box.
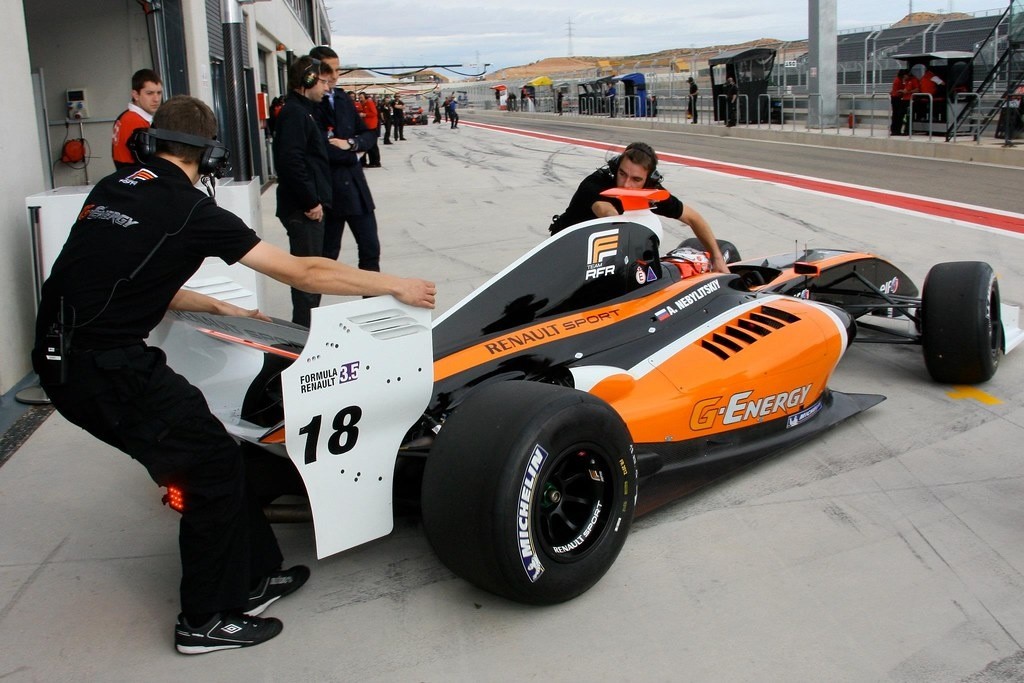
[451,95,456,98]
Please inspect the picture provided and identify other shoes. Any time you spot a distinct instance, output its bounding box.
[400,138,406,140]
[395,138,397,141]
[451,126,459,129]
[384,141,393,144]
[366,163,381,167]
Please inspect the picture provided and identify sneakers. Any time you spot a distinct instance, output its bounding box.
[175,613,283,655]
[241,565,311,617]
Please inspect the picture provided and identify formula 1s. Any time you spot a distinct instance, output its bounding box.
[145,183,1004,607]
[389,106,428,125]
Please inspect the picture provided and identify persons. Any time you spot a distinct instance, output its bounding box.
[111,69,164,171]
[889,64,945,136]
[347,91,463,167]
[605,82,617,118]
[275,56,334,327]
[686,77,699,123]
[724,77,738,127]
[495,85,529,112]
[32,95,435,655]
[308,45,381,300]
[548,142,732,274]
[268,95,287,147]
[556,90,564,116]
